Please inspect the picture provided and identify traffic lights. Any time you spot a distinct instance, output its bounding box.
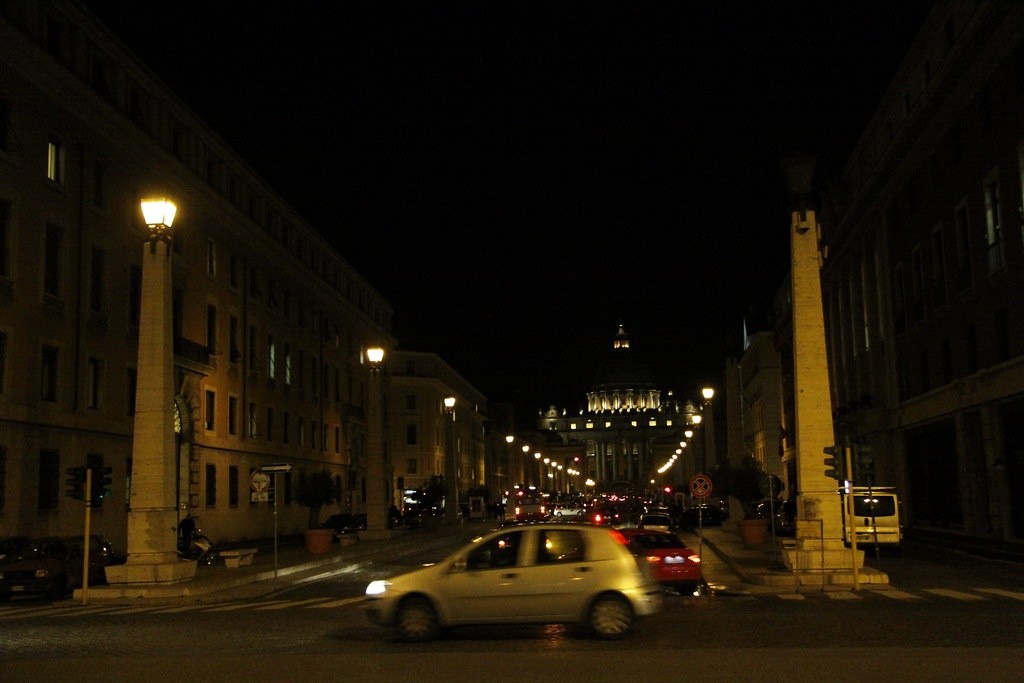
[824,443,843,478]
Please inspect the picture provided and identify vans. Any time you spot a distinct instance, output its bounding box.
[839,486,904,550]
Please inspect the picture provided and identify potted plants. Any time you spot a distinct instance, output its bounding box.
[720,452,784,545]
[415,474,449,531]
[295,470,340,554]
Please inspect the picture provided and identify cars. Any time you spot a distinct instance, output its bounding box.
[367,490,730,642]
[0,535,118,604]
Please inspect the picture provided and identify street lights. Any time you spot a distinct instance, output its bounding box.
[105,194,197,587]
[503,436,517,521]
[702,387,716,474]
[358,348,403,541]
[442,398,465,528]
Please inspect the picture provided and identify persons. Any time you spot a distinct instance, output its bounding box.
[178,514,196,558]
[388,505,403,524]
[462,504,471,519]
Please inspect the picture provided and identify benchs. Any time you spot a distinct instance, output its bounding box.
[220,547,258,567]
[337,533,357,546]
